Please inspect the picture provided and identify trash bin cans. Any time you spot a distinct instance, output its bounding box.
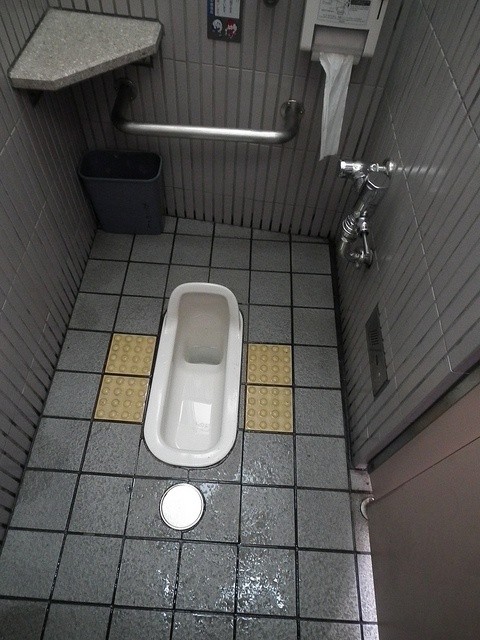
[78,149,165,235]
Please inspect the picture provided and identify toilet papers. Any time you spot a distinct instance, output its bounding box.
[310,26,367,162]
